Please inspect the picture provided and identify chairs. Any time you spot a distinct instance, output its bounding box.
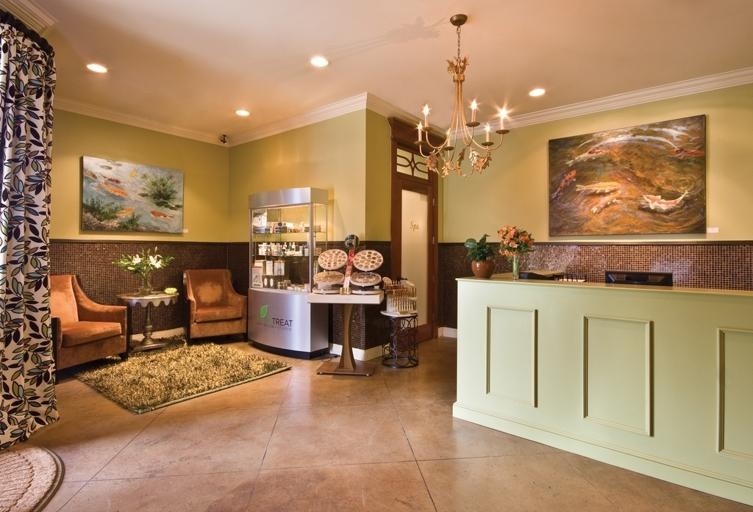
[183,269,247,347]
[49,274,130,384]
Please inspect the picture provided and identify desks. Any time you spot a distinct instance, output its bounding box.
[308,288,385,377]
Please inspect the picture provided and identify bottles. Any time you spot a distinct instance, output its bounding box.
[270,242,307,256]
[282,223,287,232]
[279,279,291,289]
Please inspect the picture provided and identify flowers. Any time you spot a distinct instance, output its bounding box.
[498,226,534,257]
[111,244,176,288]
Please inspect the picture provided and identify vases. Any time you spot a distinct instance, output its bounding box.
[138,275,152,295]
[512,254,520,280]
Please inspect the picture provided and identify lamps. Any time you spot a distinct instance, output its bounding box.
[414,14,511,179]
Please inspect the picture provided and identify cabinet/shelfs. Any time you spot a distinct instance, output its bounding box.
[247,187,329,359]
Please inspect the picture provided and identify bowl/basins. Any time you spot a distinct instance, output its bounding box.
[163,288,177,294]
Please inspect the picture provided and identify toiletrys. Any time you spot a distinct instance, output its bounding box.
[257,242,308,292]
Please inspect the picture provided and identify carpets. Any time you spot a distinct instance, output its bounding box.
[69,341,292,415]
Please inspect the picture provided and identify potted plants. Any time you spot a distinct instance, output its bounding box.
[464,233,496,279]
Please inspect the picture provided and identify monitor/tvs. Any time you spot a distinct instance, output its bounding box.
[605,270,673,287]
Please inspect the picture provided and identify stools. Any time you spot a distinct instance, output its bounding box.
[380,310,419,369]
